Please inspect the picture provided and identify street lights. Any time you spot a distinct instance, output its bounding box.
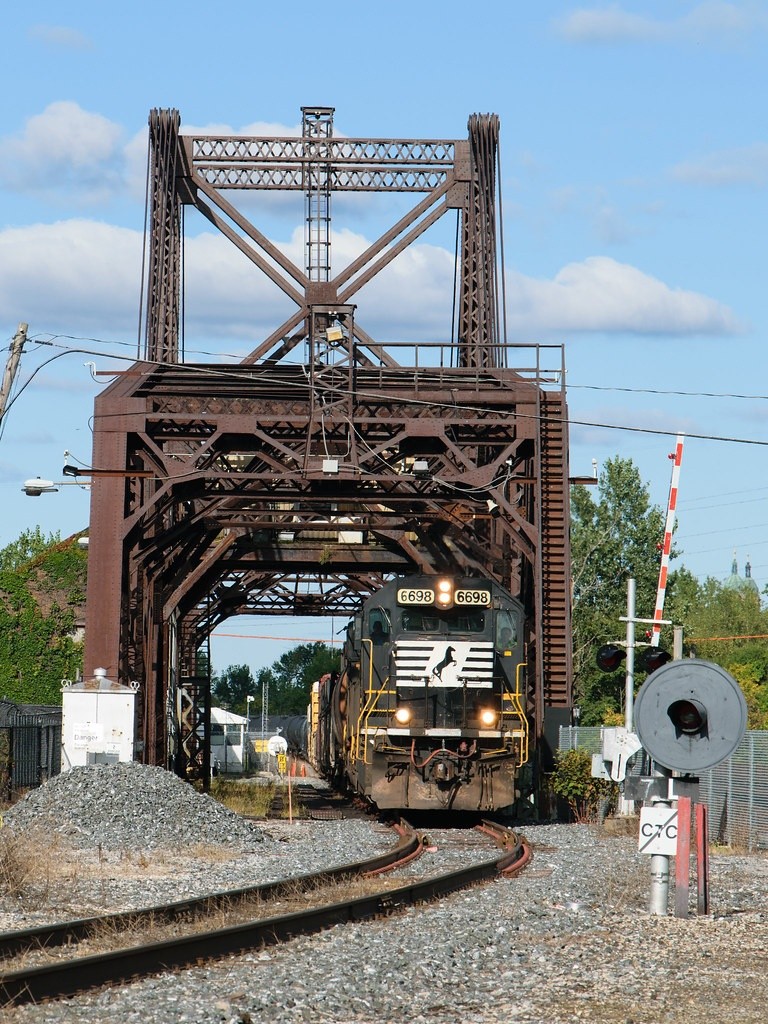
[19,476,91,497]
[245,695,256,732]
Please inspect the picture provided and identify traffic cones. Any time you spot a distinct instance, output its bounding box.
[291,763,297,777]
[301,764,306,777]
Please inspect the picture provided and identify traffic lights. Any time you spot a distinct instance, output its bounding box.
[595,644,626,672]
[640,646,672,675]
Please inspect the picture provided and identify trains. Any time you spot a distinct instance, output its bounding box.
[236,571,529,816]
